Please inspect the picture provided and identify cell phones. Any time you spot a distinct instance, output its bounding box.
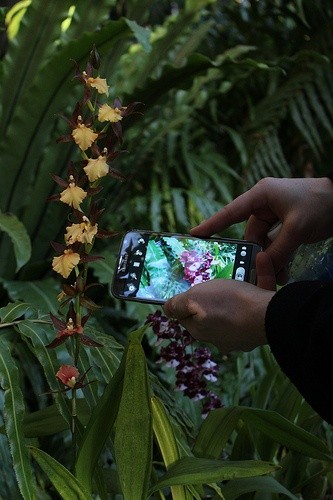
[112,229,263,305]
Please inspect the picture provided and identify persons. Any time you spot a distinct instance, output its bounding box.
[166,168,333,427]
